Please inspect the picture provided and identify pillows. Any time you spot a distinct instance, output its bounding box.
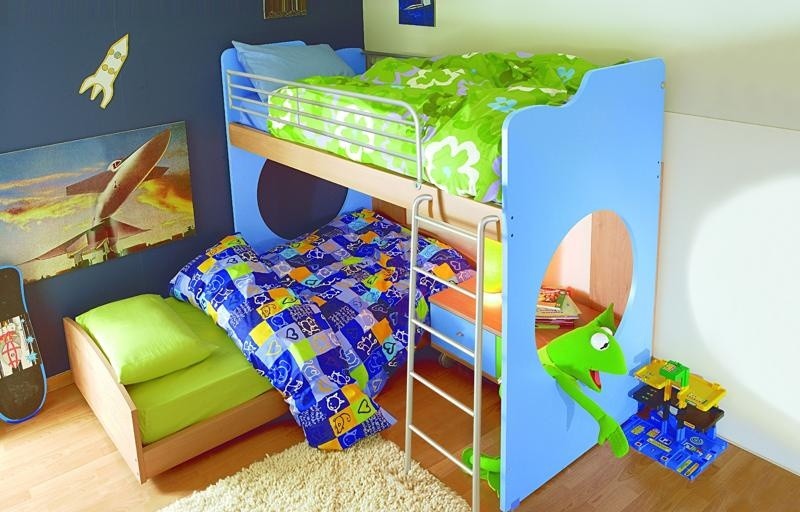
[231,39,357,103]
[74,292,220,385]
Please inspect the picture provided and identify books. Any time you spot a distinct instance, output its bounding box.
[500,289,583,332]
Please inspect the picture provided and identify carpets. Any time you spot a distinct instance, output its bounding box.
[155,431,472,512]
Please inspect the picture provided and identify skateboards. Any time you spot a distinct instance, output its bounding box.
[1,266,45,422]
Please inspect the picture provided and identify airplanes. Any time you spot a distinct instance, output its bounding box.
[15,127,174,267]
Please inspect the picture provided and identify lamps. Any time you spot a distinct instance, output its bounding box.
[482,236,505,306]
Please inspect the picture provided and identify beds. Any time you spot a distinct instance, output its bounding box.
[63,206,478,485]
[220,36,668,512]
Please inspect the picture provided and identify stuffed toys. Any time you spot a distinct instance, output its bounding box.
[461,302,630,503]
